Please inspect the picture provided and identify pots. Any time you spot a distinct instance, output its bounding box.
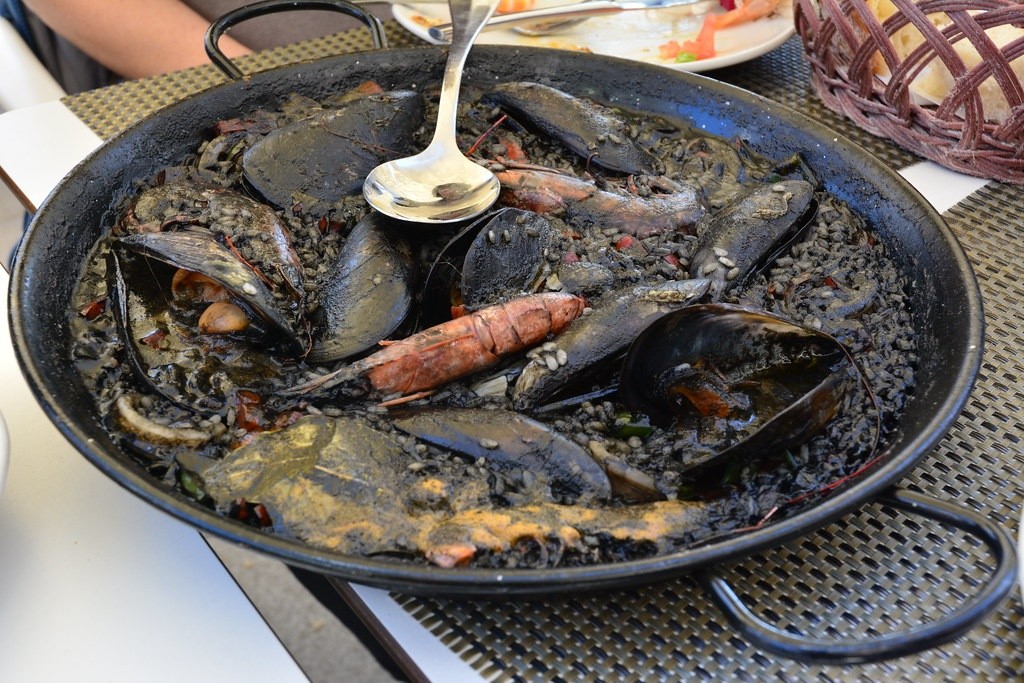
[5,0,1019,667]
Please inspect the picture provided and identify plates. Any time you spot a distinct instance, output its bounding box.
[391,0,797,74]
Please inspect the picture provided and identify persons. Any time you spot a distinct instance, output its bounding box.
[0,0,395,80]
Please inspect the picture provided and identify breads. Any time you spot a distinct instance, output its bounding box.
[815,0,1024,126]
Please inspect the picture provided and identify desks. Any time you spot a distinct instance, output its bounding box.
[0,19,1024,682]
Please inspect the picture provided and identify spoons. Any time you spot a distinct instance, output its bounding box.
[362,0,504,224]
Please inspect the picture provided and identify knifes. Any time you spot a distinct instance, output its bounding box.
[428,0,702,42]
